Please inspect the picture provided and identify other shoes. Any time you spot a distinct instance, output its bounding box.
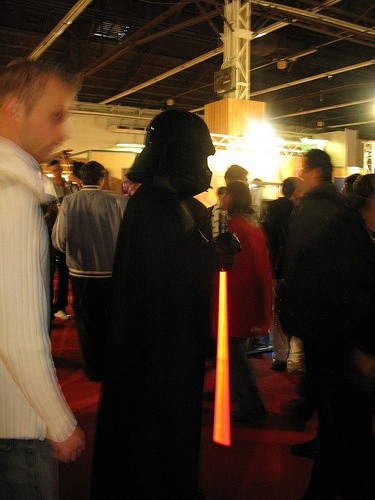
[271,358,284,371]
[54,310,71,320]
[291,436,320,459]
[230,409,267,422]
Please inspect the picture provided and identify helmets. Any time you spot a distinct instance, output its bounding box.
[143,108,214,195]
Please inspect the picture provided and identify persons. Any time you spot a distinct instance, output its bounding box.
[281,148,347,458]
[51,160,135,382]
[303,173,375,500]
[46,158,86,320]
[92,109,242,500]
[0,55,85,500]
[266,177,305,375]
[212,165,249,239]
[209,181,274,426]
